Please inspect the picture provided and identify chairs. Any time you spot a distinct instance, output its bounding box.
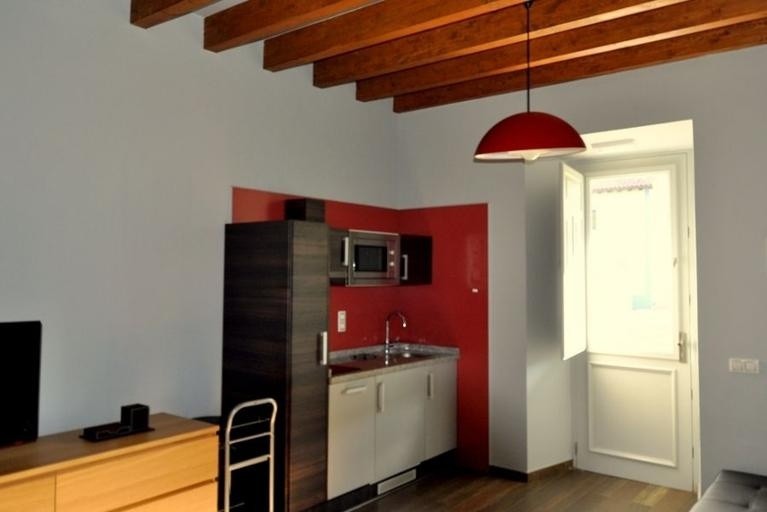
[688,470,767,511]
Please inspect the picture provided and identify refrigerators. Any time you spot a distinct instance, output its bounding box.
[219,218,331,512]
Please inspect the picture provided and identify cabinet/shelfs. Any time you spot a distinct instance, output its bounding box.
[0,412,220,512]
[327,355,458,501]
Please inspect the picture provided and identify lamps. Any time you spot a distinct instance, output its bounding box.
[473,0,587,164]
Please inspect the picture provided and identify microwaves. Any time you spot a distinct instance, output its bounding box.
[345,231,400,287]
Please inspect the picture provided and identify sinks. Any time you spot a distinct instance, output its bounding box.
[389,347,434,359]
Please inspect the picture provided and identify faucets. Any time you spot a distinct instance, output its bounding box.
[384,308,408,367]
